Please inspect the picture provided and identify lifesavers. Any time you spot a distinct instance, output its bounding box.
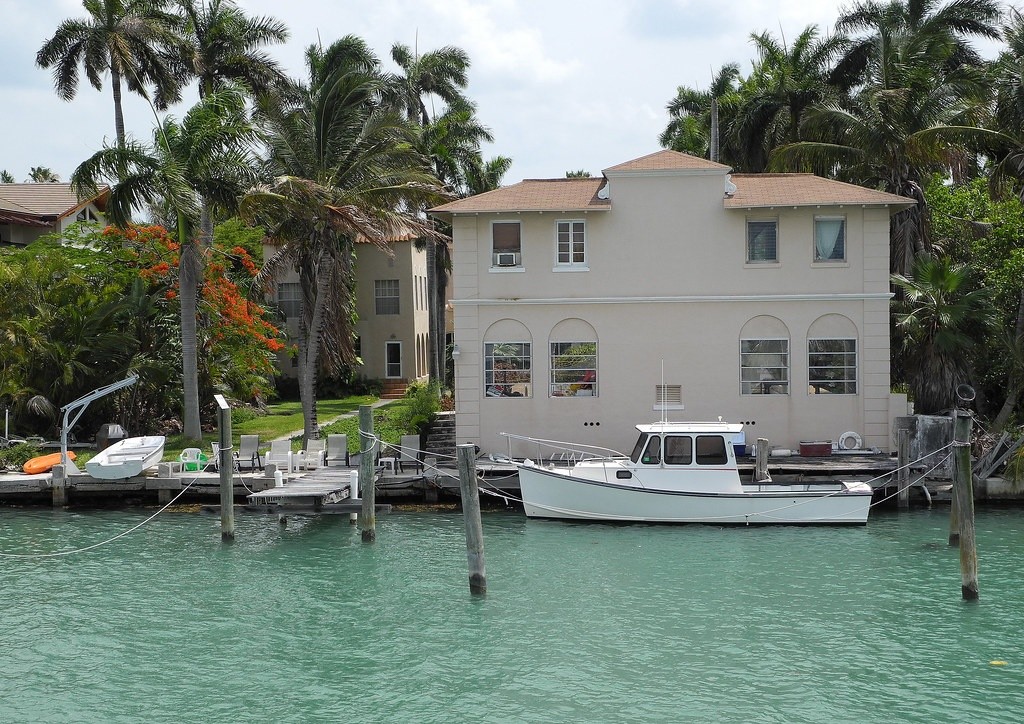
[838,431,863,450]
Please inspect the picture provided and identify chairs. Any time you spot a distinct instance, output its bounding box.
[395,434,423,475]
[373,433,381,466]
[265,440,294,474]
[296,439,326,473]
[324,434,349,467]
[180,448,202,473]
[232,435,262,474]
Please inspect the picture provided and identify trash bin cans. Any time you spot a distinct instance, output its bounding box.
[96,423,128,454]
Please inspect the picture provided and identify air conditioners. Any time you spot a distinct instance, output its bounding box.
[496,253,516,266]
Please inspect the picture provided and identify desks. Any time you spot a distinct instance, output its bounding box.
[378,458,401,474]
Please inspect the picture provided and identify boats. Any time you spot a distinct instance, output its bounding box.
[516,357,873,525]
[25,450,76,474]
[85,434,166,479]
